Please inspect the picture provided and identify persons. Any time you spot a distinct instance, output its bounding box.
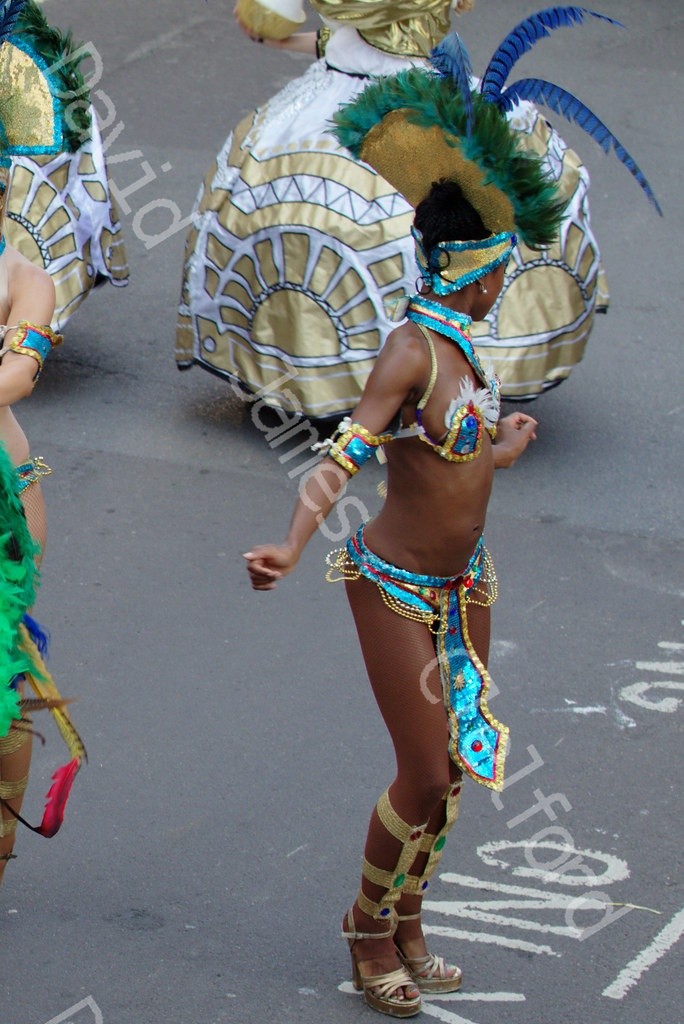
[0,0,132,334]
[174,0,611,420]
[0,125,92,882]
[242,176,538,1017]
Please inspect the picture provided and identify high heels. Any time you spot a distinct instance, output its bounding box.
[340,902,433,1018]
[395,912,461,994]
